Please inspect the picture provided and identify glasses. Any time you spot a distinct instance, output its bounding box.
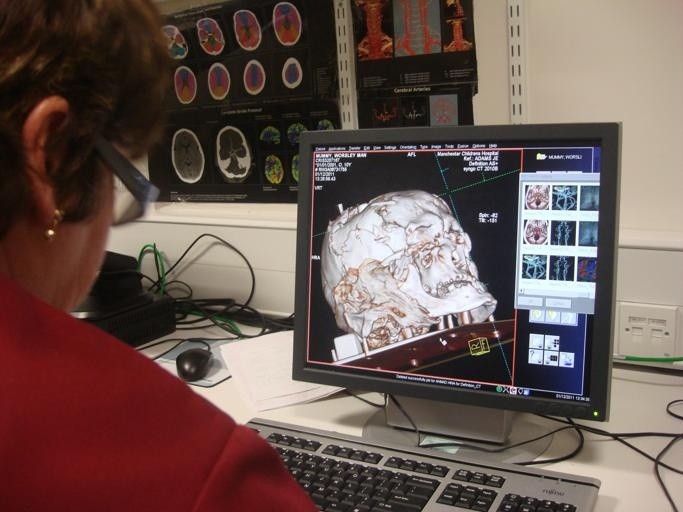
[87,124,159,226]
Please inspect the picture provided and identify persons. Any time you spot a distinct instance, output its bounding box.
[0,0,323,511]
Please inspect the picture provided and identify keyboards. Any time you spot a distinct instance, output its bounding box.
[238,418,601,512]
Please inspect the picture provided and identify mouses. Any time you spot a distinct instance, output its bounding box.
[176,348,213,380]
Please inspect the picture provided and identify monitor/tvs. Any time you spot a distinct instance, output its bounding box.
[293,121,626,462]
